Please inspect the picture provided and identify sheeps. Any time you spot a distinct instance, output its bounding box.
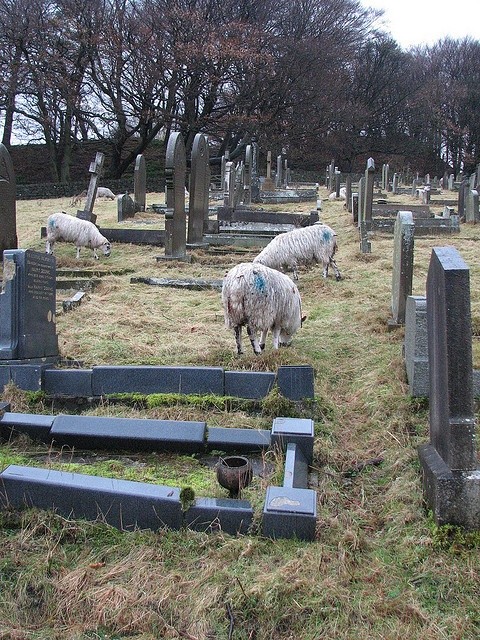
[45,213,111,260]
[252,224,341,280]
[223,262,308,355]
[96,187,116,201]
